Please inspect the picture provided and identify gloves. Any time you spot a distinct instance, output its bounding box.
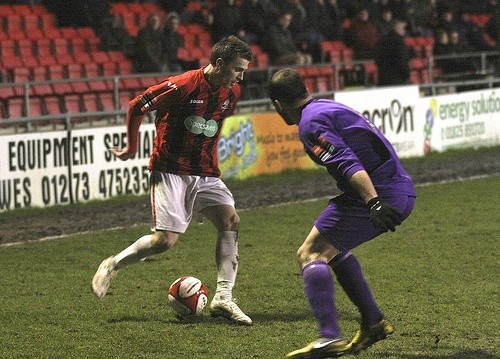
[366,196,402,233]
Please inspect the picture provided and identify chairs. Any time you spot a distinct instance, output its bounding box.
[0,0,500,135]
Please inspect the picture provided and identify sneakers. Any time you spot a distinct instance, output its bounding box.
[209,299,252,324]
[92,256,117,300]
[285,338,351,358]
[348,318,395,353]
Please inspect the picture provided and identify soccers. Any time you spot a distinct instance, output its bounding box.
[168,276,208,315]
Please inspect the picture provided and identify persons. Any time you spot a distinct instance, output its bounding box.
[91,35,253,325]
[98,0,500,90]
[269,68,417,358]
